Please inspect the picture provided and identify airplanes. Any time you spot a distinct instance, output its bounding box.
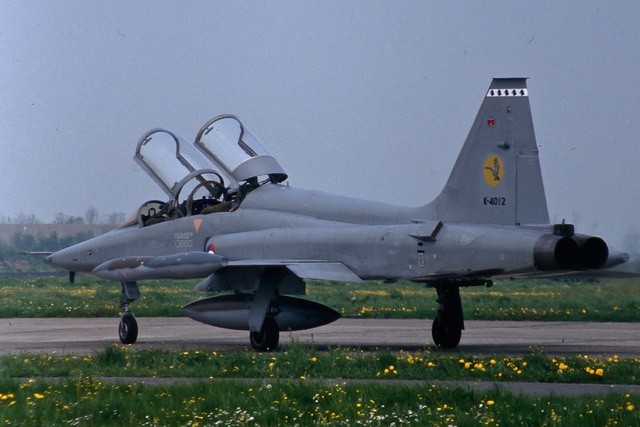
[45,77,630,351]
